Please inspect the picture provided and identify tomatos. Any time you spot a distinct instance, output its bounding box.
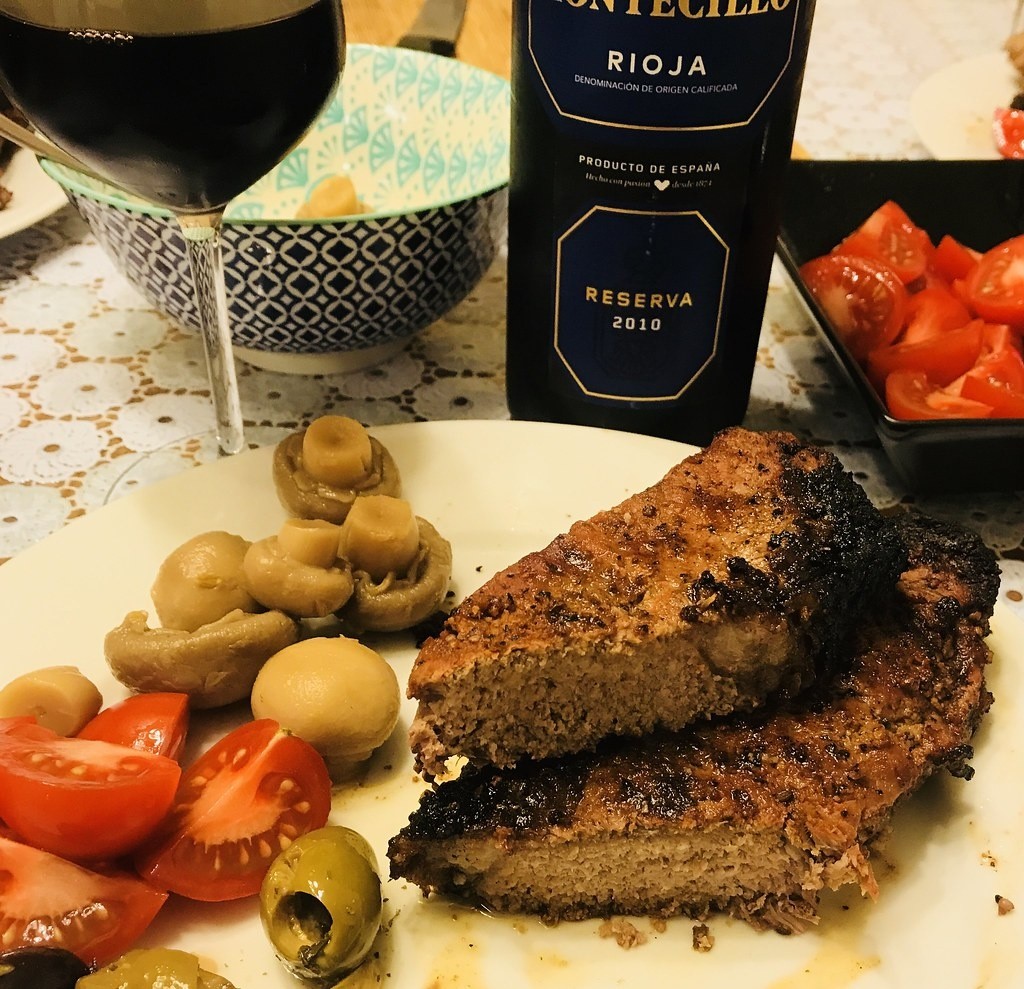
[1,699,331,969]
[803,195,1024,424]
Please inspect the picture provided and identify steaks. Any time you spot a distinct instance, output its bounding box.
[390,419,1000,938]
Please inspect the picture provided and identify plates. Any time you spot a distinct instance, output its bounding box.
[0,420,1023,988]
[0,124,73,234]
[774,158,1022,503]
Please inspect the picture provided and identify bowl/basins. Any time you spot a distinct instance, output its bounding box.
[33,44,508,374]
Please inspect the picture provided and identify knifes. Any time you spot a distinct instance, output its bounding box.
[393,1,470,60]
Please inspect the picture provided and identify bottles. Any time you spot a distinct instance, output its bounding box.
[504,0,816,447]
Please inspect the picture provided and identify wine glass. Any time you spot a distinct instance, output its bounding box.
[0,2,342,513]
